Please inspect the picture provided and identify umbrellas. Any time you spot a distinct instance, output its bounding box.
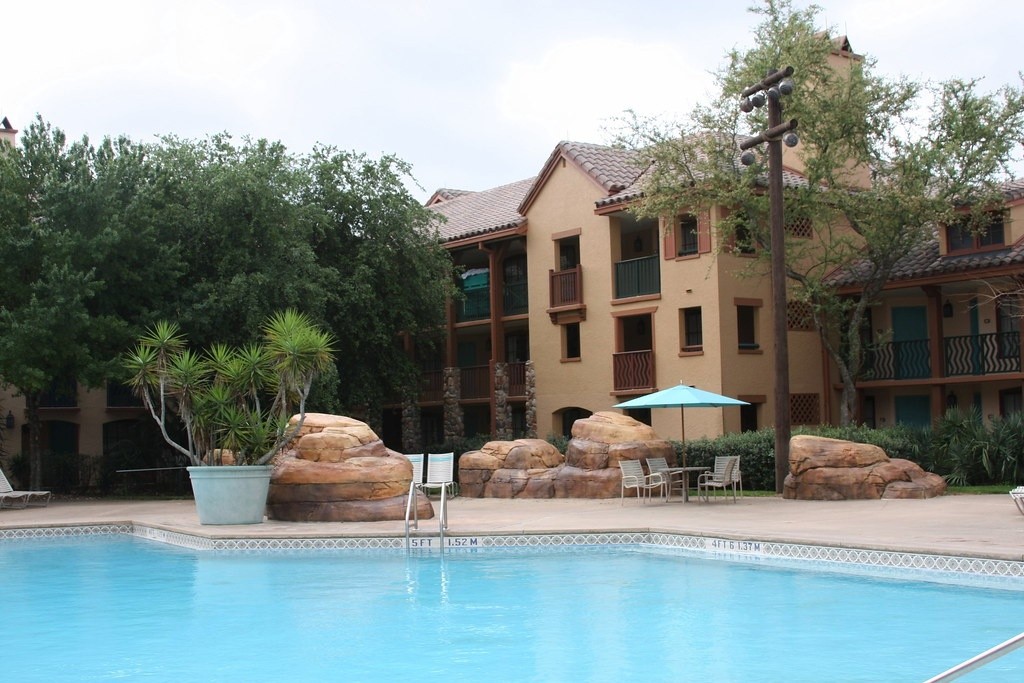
[612,384,751,468]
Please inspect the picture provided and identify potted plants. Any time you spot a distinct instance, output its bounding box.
[121,316,339,524]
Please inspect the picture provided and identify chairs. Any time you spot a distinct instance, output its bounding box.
[423,452,459,499]
[646,457,690,502]
[705,454,742,502]
[404,454,424,492]
[697,458,738,507]
[0,493,26,509]
[0,469,51,507]
[618,459,664,506]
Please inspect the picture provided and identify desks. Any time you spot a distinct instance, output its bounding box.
[659,467,711,502]
